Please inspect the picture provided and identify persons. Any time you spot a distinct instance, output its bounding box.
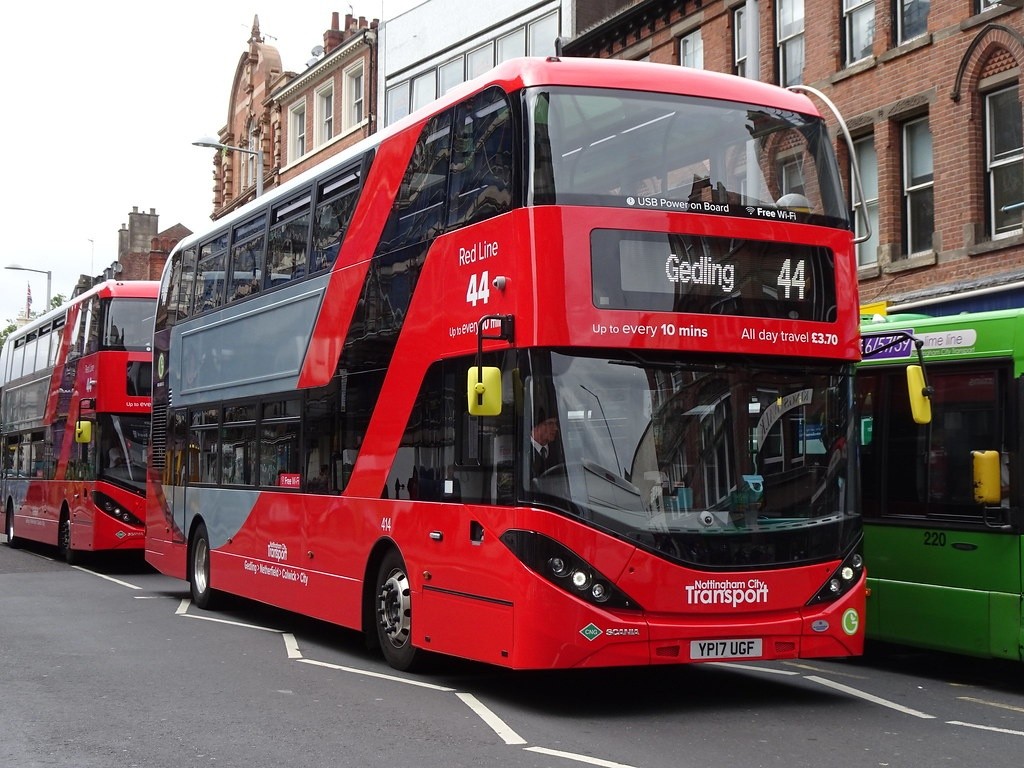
[530,404,565,478]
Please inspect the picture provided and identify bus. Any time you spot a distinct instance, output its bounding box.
[144,54,935,676]
[0,278,207,566]
[861,307,1024,667]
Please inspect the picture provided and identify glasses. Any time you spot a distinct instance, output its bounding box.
[542,421,561,428]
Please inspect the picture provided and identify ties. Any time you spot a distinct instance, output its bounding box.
[540,447,547,459]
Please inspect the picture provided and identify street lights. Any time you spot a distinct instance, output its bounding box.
[192,137,263,200]
[5,264,52,314]
